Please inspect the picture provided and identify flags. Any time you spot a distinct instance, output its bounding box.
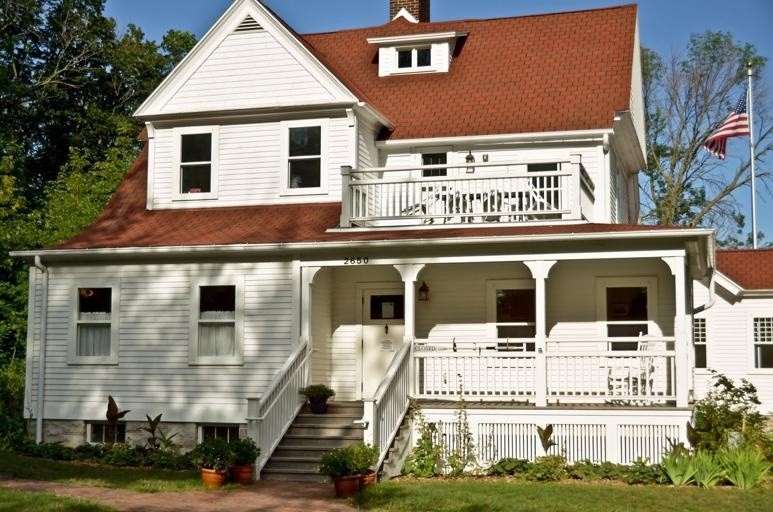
[703,87,750,160]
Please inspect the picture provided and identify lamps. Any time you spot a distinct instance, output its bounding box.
[466,150,475,173]
[419,282,430,300]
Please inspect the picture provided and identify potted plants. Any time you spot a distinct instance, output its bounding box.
[311,444,364,498]
[356,442,379,490]
[298,384,336,414]
[190,437,235,486]
[228,436,260,485]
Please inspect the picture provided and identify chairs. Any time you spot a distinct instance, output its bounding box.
[402,182,558,224]
[600,331,656,395]
